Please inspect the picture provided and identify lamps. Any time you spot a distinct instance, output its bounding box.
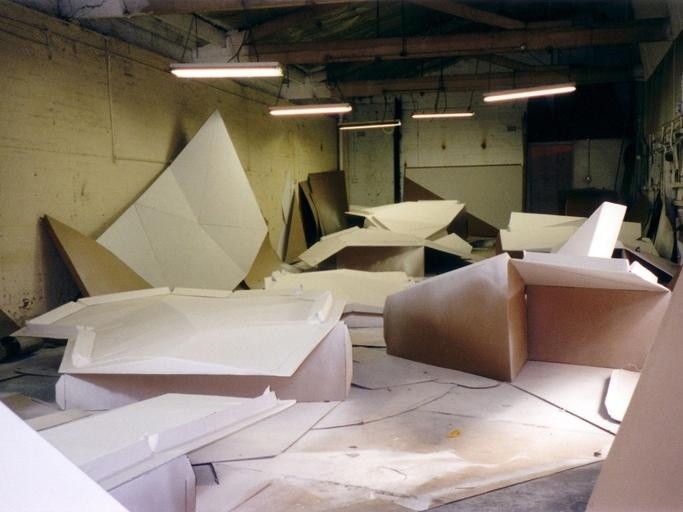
[168,10,285,80]
[406,85,476,119]
[269,62,353,115]
[337,99,401,130]
[483,53,575,102]
